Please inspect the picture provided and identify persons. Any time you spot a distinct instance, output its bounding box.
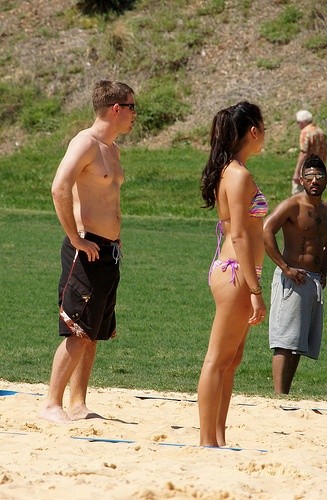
[196,98,269,447]
[262,154,327,400]
[37,79,138,427]
[292,109,326,197]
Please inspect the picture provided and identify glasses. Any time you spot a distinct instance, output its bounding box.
[108,103,134,111]
[303,174,326,181]
